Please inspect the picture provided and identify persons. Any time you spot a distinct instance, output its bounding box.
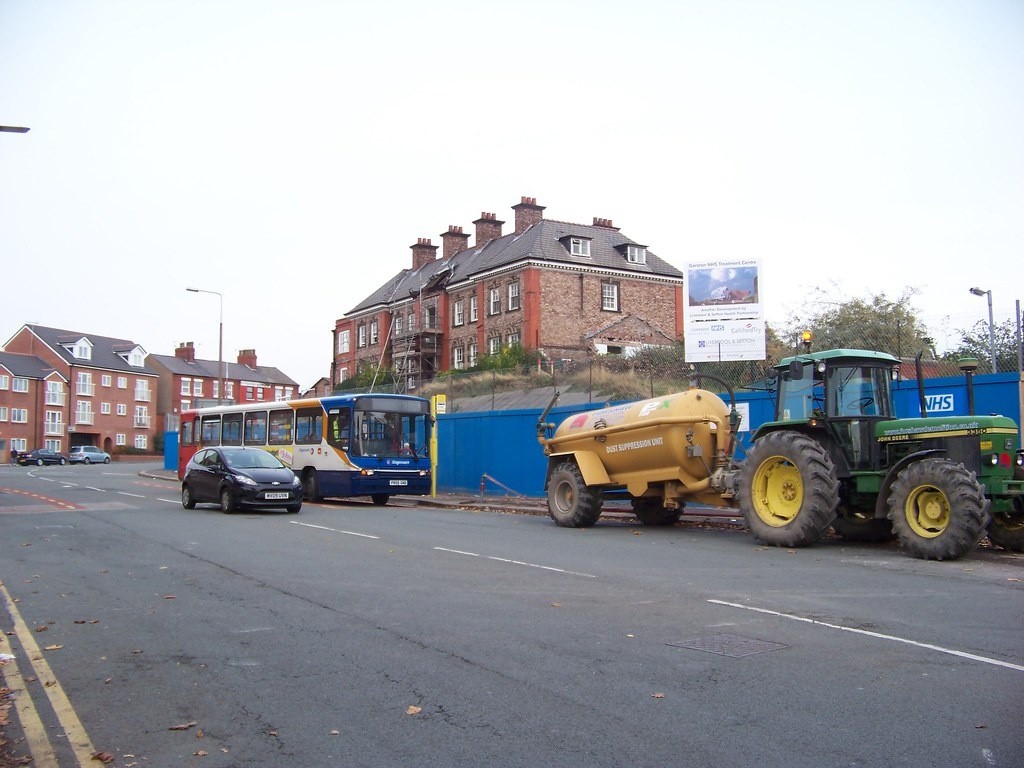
[341,425,349,443]
[10,448,18,466]
[303,433,317,440]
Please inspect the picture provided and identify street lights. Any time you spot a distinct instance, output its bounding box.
[187,288,223,405]
[969,288,997,374]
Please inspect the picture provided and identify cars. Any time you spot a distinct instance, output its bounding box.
[19,448,67,466]
[68,445,112,465]
[179,445,304,514]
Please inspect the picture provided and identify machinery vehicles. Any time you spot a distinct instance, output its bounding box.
[538,328,1023,563]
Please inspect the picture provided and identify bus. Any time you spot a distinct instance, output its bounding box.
[178,393,437,508]
